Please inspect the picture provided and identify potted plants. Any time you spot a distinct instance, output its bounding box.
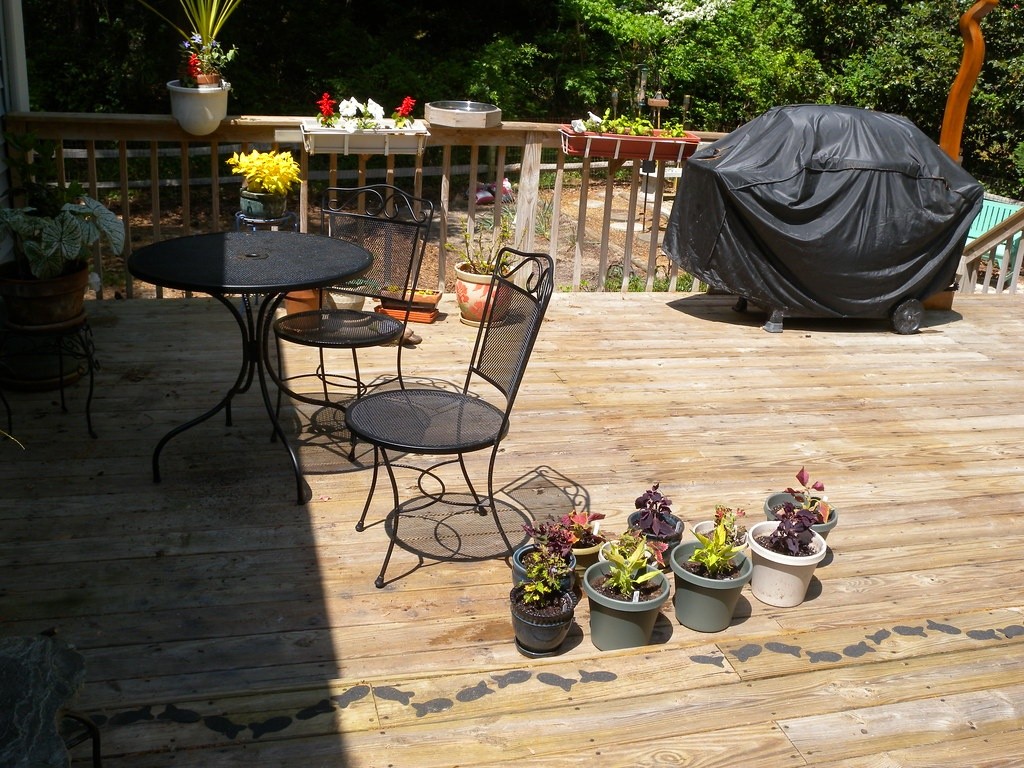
[747,502,828,609]
[692,505,750,551]
[506,541,579,658]
[668,519,753,632]
[561,108,701,161]
[764,467,838,542]
[442,214,530,326]
[626,483,685,567]
[582,535,671,652]
[0,124,127,326]
[567,510,609,580]
[226,151,304,217]
[511,517,577,587]
[599,527,667,573]
[373,285,443,323]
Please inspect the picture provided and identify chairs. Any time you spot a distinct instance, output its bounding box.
[270,182,434,460]
[344,245,556,589]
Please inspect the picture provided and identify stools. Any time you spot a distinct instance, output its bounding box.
[0,311,101,439]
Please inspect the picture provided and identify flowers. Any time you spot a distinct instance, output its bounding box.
[313,93,417,130]
[137,0,244,94]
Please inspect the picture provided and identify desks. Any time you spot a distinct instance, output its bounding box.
[128,229,373,508]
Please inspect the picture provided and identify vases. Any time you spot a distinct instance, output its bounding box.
[283,286,328,330]
[301,116,431,154]
[165,79,232,137]
